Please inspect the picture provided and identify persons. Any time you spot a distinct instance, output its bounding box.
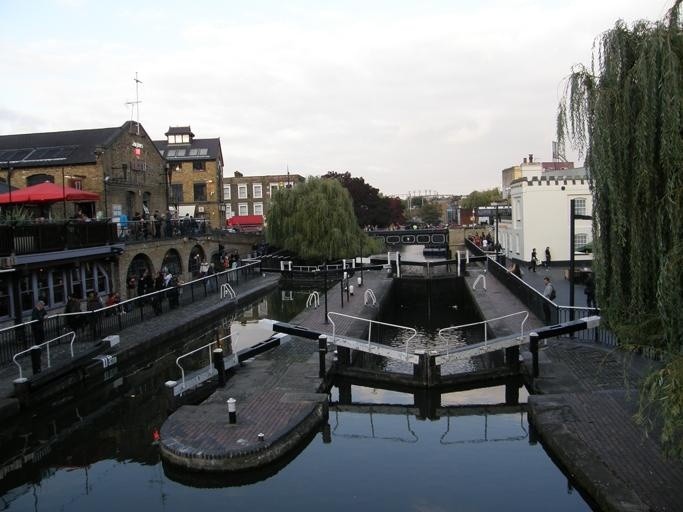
[528,248,537,272]
[32,209,240,350]
[584,280,597,314]
[545,247,551,271]
[468,231,503,252]
[506,260,522,279]
[364,222,443,232]
[542,277,553,324]
